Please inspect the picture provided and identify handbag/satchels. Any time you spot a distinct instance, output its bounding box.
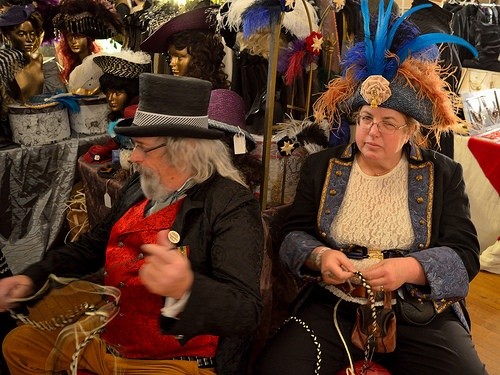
[399,296,439,326]
[352,304,396,353]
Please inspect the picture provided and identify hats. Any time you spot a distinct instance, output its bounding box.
[113,73,224,140]
[0,1,35,27]
[48,0,121,39]
[220,1,343,119]
[209,90,257,152]
[93,51,151,78]
[140,0,220,52]
[316,9,469,148]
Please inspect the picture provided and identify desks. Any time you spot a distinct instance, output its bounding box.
[78,157,302,375]
[453,133,500,275]
[0,134,110,276]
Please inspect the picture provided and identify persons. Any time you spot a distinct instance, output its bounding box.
[0,73,265,375]
[0,0,233,177]
[251,75,490,375]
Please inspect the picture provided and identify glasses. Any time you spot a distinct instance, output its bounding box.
[357,114,408,134]
[128,139,167,157]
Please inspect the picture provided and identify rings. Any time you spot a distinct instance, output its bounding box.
[380,285,385,292]
[323,271,335,278]
